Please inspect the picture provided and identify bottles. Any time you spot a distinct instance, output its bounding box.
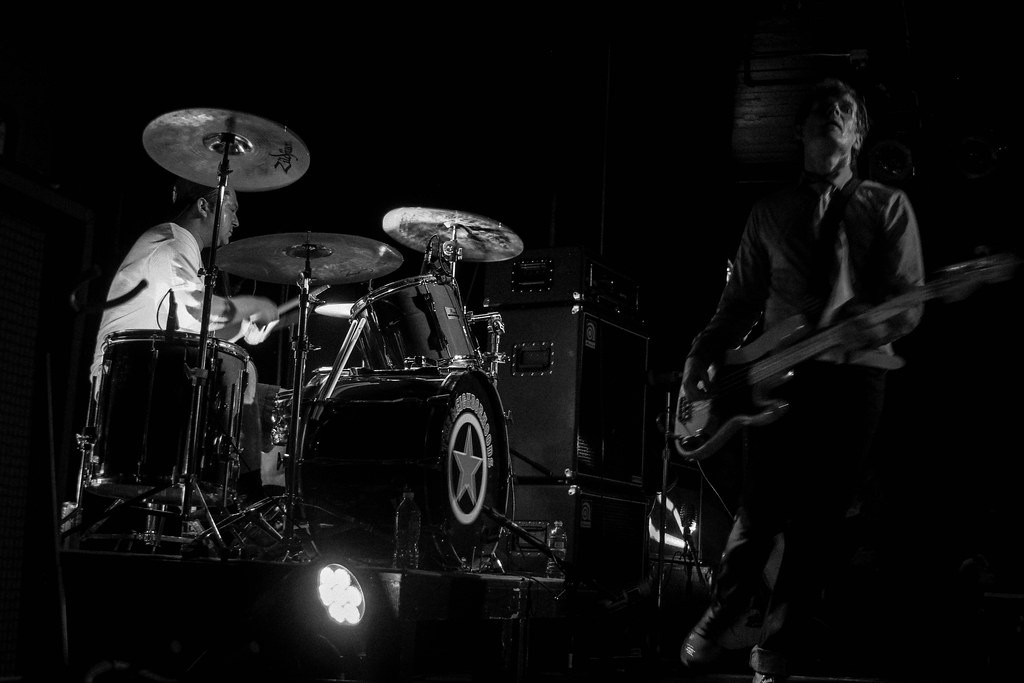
[393,493,421,569]
[546,521,568,579]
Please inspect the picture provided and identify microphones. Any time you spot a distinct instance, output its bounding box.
[421,242,433,276]
[168,292,178,330]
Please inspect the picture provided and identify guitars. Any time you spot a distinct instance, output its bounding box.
[674,253,1019,462]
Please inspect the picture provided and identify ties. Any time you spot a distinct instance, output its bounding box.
[813,183,832,237]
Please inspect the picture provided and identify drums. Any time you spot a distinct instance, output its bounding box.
[351,273,483,372]
[290,364,512,572]
[83,328,251,506]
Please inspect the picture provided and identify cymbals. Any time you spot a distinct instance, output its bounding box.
[382,206,524,263]
[142,108,313,192]
[217,233,404,286]
[314,302,356,320]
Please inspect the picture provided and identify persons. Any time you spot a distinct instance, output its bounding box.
[73,176,281,504]
[675,79,925,683]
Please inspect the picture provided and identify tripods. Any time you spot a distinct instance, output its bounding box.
[59,140,394,567]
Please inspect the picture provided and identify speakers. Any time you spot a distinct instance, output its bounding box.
[493,308,654,607]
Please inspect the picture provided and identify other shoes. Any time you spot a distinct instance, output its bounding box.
[680,592,754,672]
[750,607,812,675]
